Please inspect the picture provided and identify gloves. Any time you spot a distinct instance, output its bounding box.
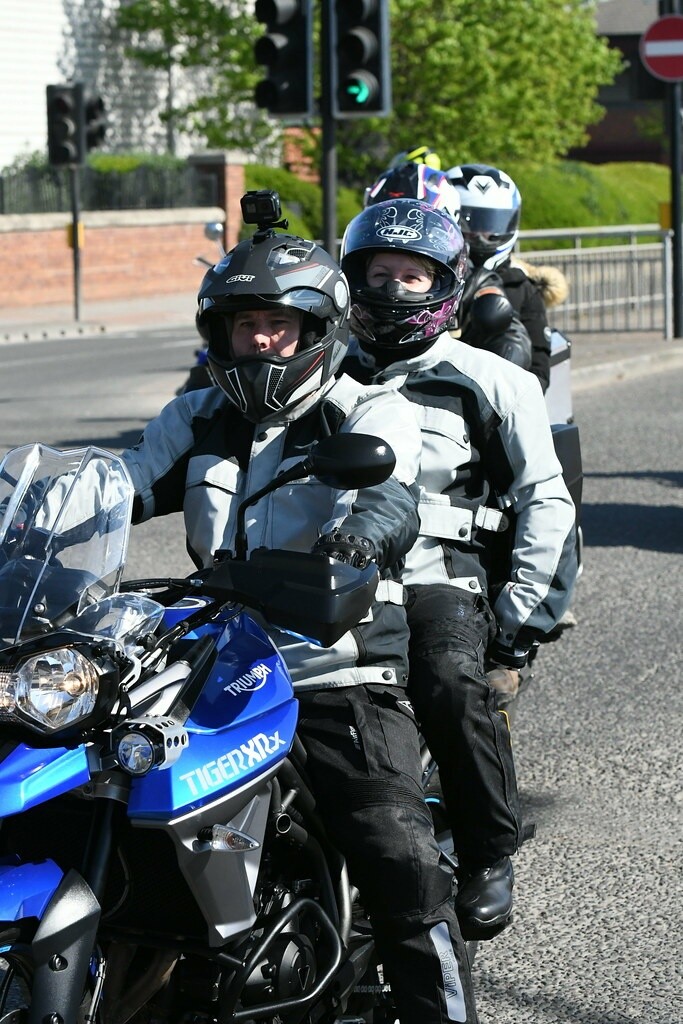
[485,667,519,710]
[309,532,376,572]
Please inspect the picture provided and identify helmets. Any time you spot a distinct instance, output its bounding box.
[338,197,467,347]
[365,165,459,230]
[445,163,522,274]
[196,235,350,425]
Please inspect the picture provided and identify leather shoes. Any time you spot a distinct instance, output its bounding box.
[455,854,514,940]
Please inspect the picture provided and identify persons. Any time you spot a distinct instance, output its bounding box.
[1,231,481,1024]
[432,166,578,629]
[343,164,531,657]
[176,199,574,938]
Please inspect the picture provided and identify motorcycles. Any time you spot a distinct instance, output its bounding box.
[2,442,423,1024]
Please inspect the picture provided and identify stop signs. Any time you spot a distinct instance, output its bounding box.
[639,16,683,84]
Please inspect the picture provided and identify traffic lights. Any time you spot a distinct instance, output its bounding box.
[253,0,312,117]
[326,0,393,119]
[83,94,106,152]
[46,84,83,169]
